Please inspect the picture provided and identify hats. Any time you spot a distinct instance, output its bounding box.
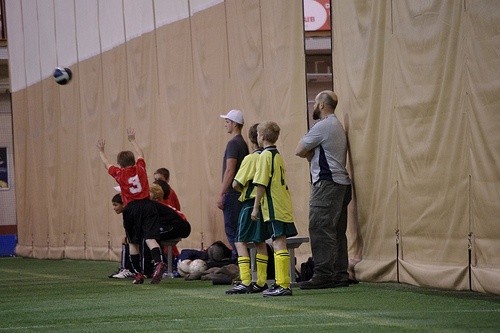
[220,109,245,124]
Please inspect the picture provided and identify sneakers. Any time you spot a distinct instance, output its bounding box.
[225,283,252,295]
[151,262,167,284]
[132,274,144,284]
[252,283,268,293]
[112,268,136,278]
[262,280,292,296]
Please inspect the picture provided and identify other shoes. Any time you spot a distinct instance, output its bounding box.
[300,277,336,289]
[335,280,350,287]
[107,267,123,278]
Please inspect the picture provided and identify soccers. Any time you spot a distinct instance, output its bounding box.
[53,66,73,86]
[177,258,207,280]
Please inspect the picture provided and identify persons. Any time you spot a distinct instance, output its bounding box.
[217,110,249,258]
[97,127,167,284]
[251,121,298,296]
[226,123,269,294]
[297,90,352,288]
[111,167,191,279]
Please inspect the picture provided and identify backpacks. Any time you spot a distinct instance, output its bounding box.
[212,264,240,286]
[301,257,315,281]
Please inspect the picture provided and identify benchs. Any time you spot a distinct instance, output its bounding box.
[246,237,309,288]
